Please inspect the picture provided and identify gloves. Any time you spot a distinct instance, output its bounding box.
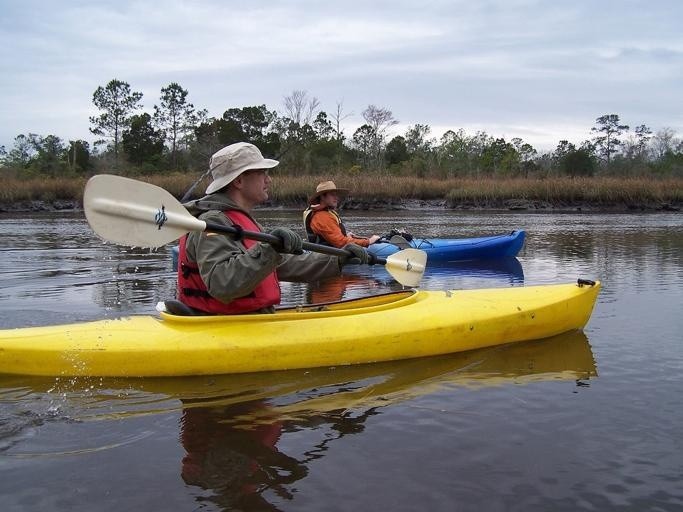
[339,243,368,265]
[270,228,304,255]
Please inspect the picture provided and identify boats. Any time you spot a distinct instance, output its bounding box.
[365,228,526,260]
[43,332,601,433]
[0,278,600,378]
[344,260,527,285]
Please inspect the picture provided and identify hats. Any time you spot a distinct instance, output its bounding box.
[204,142,280,194]
[307,181,349,205]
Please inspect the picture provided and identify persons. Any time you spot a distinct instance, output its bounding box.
[179,141,366,316]
[304,180,380,251]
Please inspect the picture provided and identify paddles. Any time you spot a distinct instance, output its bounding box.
[349,234,413,250]
[82,173,427,288]
